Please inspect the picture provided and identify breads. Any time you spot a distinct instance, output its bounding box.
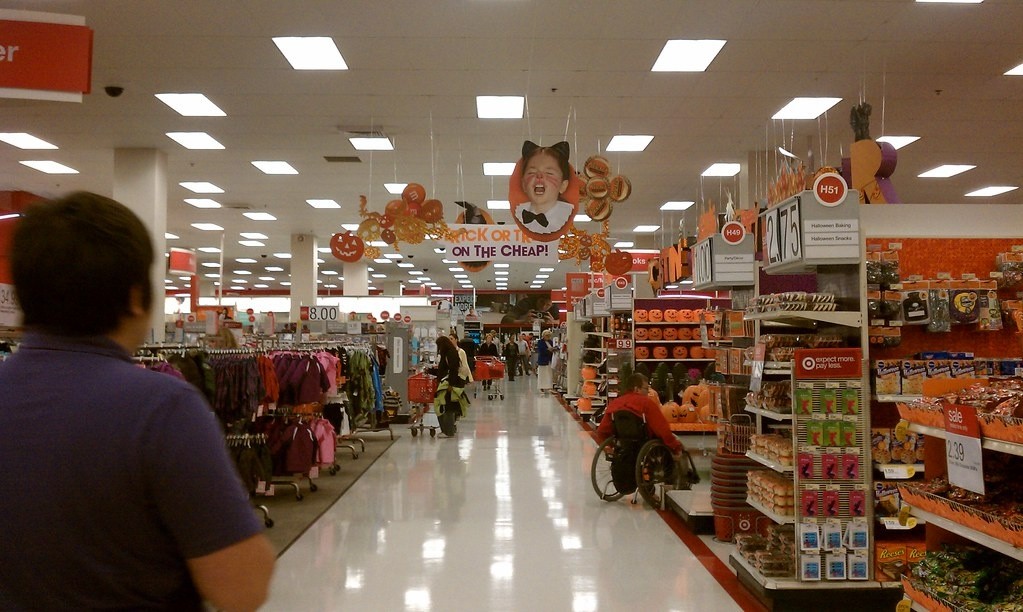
[745,379,796,517]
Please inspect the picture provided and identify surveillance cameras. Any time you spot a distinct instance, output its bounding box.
[487,280,491,282]
[261,255,266,258]
[397,260,402,263]
[104,86,124,97]
[423,269,428,271]
[439,248,445,250]
[408,255,413,258]
[525,282,529,283]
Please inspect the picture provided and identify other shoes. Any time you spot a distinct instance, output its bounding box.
[509,380,514,381]
[483,386,486,391]
[487,386,490,390]
[437,431,456,438]
[540,389,545,393]
[519,374,522,376]
[526,374,530,376]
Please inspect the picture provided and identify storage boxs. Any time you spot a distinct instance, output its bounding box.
[900,360,926,395]
[891,429,916,464]
[869,359,900,395]
[876,541,907,582]
[871,428,891,464]
[917,351,951,378]
[873,481,915,518]
[916,433,924,464]
[947,352,976,379]
[904,539,926,578]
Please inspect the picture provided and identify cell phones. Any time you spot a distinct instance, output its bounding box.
[531,311,548,319]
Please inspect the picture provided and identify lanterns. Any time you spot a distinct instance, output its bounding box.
[635,328,723,340]
[650,309,716,323]
[636,344,717,358]
[577,365,597,412]
[694,308,705,323]
[648,382,716,423]
[634,309,647,322]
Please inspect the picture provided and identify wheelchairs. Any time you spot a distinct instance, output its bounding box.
[591,403,700,511]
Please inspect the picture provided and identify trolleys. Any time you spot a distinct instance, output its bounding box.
[406,372,439,437]
[473,356,505,400]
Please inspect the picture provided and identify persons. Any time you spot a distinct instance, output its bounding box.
[281,322,310,334]
[460,331,478,375]
[424,334,474,438]
[479,328,500,391]
[0,191,275,612]
[514,141,574,235]
[465,307,477,321]
[172,297,185,313]
[597,373,693,491]
[537,329,558,394]
[504,332,534,382]
[217,308,233,320]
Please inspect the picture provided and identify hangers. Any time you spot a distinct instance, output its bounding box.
[139,340,385,449]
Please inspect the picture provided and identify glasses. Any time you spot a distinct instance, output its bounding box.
[634,386,650,391]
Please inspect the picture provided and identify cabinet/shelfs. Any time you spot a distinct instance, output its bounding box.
[563,189,1023,612]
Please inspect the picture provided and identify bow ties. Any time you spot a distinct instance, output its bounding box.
[522,209,548,228]
